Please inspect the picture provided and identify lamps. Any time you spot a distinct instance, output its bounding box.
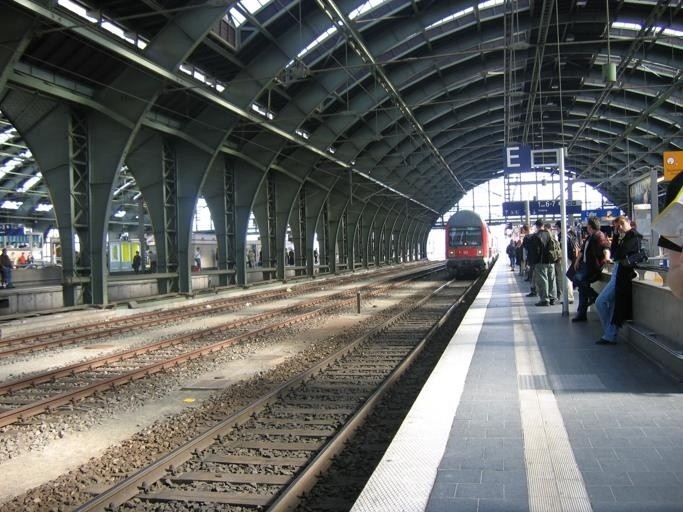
[600,1,618,85]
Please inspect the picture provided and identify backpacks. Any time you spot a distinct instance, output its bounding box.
[567,233,581,260]
[546,229,563,262]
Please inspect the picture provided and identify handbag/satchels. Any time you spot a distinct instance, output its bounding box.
[621,239,649,269]
[507,245,513,255]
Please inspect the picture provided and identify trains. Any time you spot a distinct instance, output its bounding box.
[445,210,499,280]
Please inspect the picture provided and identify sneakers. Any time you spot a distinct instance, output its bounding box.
[524,278,531,282]
[586,296,598,308]
[560,301,574,305]
[534,299,556,306]
[595,338,618,345]
[526,291,537,297]
[571,318,587,323]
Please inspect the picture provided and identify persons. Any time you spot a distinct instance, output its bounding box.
[0,248,34,289]
[506,215,643,345]
[285,248,294,265]
[657,171,683,298]
[193,247,202,272]
[314,247,319,264]
[133,251,157,273]
[248,248,256,267]
